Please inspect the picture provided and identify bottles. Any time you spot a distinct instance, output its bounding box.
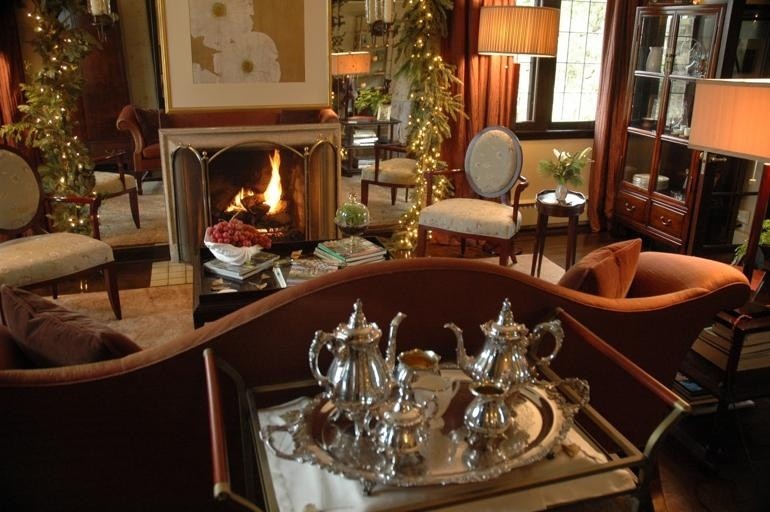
[343,81,355,119]
[333,194,370,235]
[645,46,665,74]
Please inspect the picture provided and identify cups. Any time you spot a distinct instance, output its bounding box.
[459,379,516,466]
[678,123,691,137]
[643,117,657,131]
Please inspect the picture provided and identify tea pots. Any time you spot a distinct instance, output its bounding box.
[307,299,406,411]
[358,382,439,457]
[444,298,565,399]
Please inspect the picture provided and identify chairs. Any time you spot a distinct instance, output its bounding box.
[19,129,150,238]
[419,125,529,267]
[2,146,125,324]
[117,103,163,192]
[361,141,419,209]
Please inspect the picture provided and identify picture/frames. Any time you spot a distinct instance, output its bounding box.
[155,1,332,116]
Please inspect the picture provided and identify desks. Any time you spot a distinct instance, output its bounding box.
[529,188,587,280]
[340,114,402,182]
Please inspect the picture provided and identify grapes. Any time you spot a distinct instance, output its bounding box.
[206,219,273,249]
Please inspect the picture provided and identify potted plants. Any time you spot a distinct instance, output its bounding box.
[538,144,596,202]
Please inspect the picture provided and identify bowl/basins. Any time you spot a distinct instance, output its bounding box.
[204,226,262,266]
[634,173,671,190]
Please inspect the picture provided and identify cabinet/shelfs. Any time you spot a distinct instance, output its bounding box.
[3,1,131,168]
[605,2,739,255]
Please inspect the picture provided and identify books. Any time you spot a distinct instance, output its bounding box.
[202,236,387,288]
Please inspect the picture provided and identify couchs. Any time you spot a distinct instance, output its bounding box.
[2,252,751,510]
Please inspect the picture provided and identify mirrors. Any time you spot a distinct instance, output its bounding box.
[1,2,168,277]
[332,1,440,234]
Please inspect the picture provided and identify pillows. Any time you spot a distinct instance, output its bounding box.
[556,236,642,303]
[2,282,146,372]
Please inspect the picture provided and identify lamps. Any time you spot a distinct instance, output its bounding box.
[686,73,770,167]
[365,0,394,49]
[476,6,563,59]
[330,50,372,119]
[85,0,120,44]
